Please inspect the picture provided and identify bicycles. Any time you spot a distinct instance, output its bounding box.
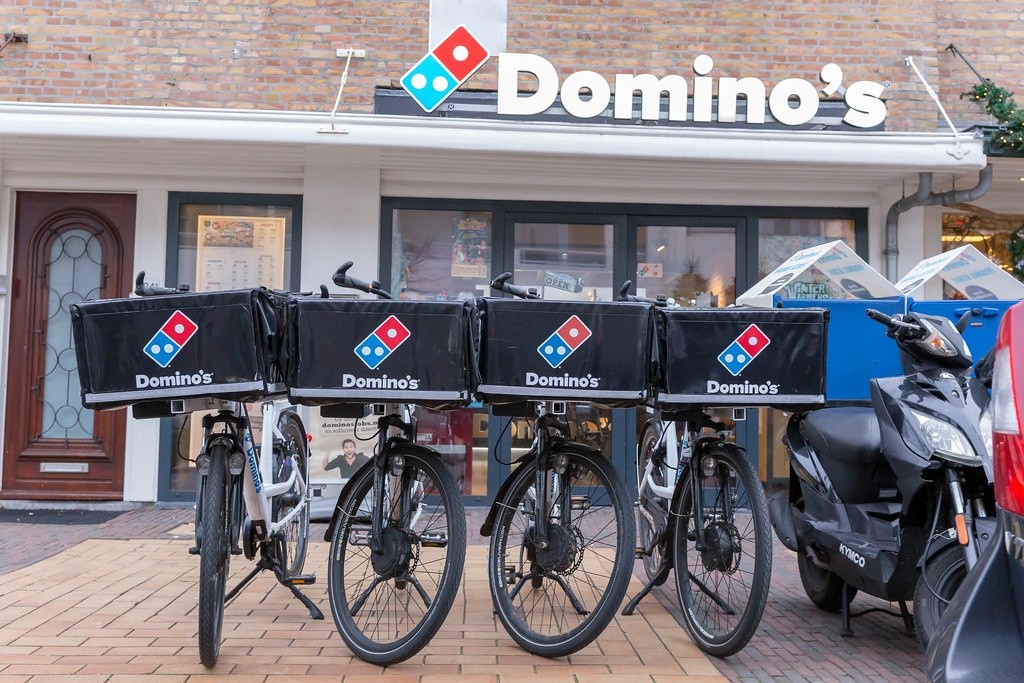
[618,281,774,659]
[129,271,325,671]
[321,260,466,668]
[478,270,636,661]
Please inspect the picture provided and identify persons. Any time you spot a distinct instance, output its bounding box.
[323,439,369,478]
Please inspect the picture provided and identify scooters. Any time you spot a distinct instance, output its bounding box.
[767,307,998,653]
